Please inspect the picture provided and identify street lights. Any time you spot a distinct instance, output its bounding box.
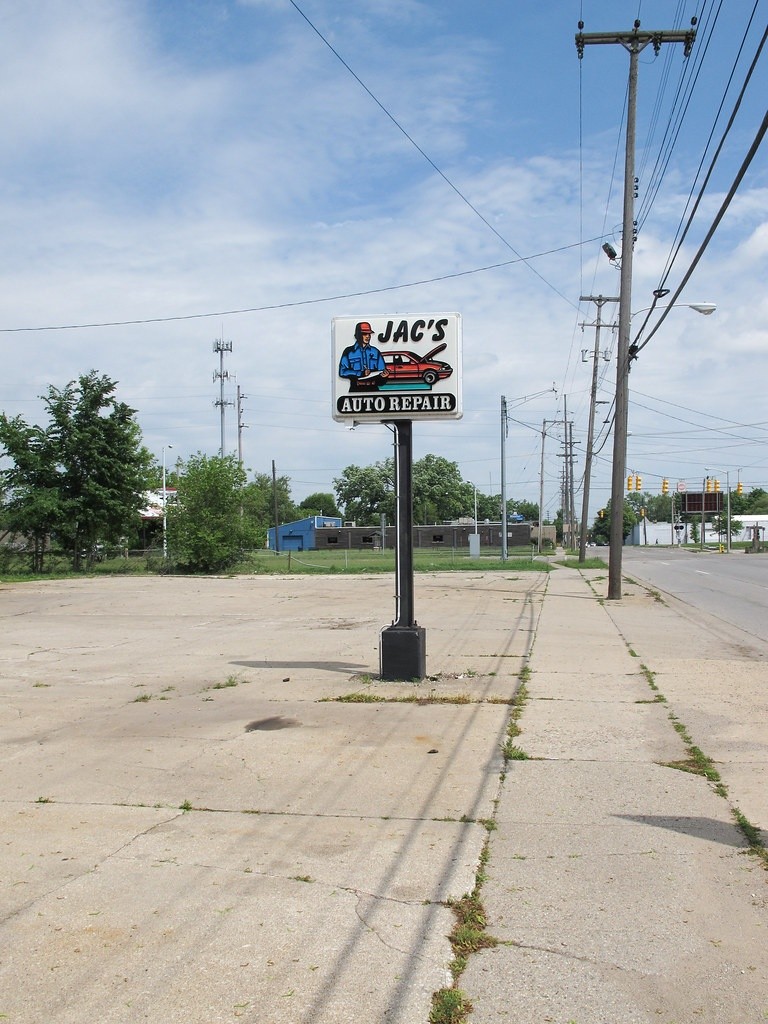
[704,467,730,553]
[644,505,647,545]
[467,480,477,534]
[608,302,716,600]
[579,349,612,563]
[163,445,173,557]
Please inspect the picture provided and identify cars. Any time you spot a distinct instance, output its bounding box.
[80,540,115,561]
[590,542,596,547]
[596,542,608,546]
[579,541,588,548]
